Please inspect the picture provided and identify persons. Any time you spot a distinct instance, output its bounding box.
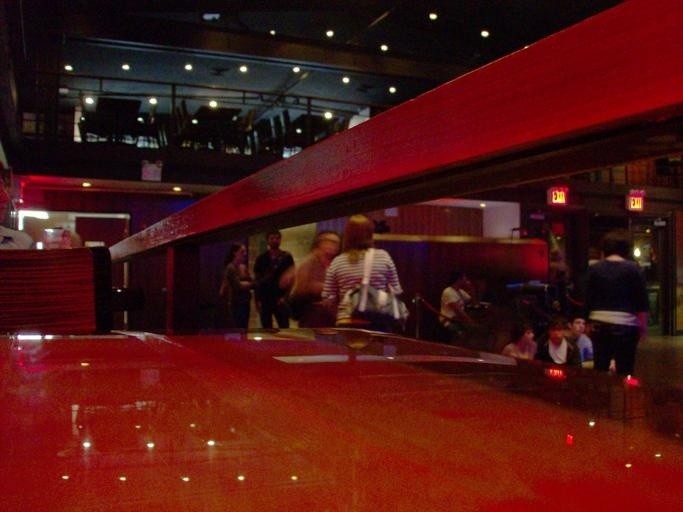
[549,247,573,310]
[502,322,538,360]
[322,213,402,328]
[256,230,295,329]
[535,317,581,366]
[279,231,341,328]
[440,262,477,333]
[219,243,255,327]
[568,315,594,369]
[585,231,651,375]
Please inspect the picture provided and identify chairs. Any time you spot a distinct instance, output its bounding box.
[510,282,551,341]
[414,291,476,341]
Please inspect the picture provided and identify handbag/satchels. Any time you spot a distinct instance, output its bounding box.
[348,247,408,322]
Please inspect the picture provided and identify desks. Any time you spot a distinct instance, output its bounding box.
[0,326,683,509]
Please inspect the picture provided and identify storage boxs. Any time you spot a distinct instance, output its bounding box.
[3,244,113,334]
